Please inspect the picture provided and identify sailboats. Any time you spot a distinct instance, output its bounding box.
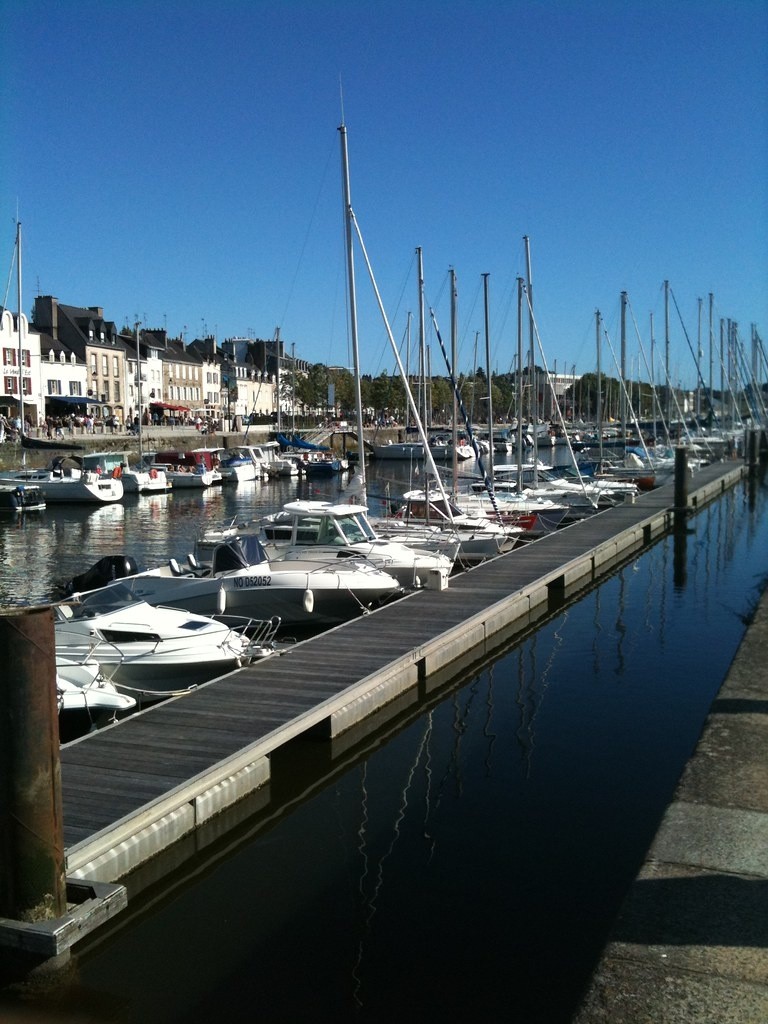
[243,326,348,476]
[194,68,505,587]
[0,202,124,502]
[46,322,173,494]
[369,236,768,464]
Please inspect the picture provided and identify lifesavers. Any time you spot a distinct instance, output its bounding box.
[112,467,122,479]
[150,468,158,479]
[460,439,464,445]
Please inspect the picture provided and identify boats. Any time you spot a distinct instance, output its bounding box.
[144,447,232,488]
[221,444,280,484]
[63,514,401,635]
[46,583,282,740]
[199,499,455,589]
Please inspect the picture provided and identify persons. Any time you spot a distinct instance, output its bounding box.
[231,416,238,432]
[147,412,218,435]
[41,412,118,436]
[0,412,32,442]
[125,414,140,436]
[364,413,398,427]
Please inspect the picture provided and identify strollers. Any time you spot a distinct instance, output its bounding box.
[129,422,142,436]
[5,426,19,443]
[201,422,209,434]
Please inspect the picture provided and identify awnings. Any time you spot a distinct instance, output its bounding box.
[153,403,191,410]
[0,396,29,408]
[50,397,108,405]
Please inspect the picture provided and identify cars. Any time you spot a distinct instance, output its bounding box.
[250,413,265,417]
[241,415,251,423]
